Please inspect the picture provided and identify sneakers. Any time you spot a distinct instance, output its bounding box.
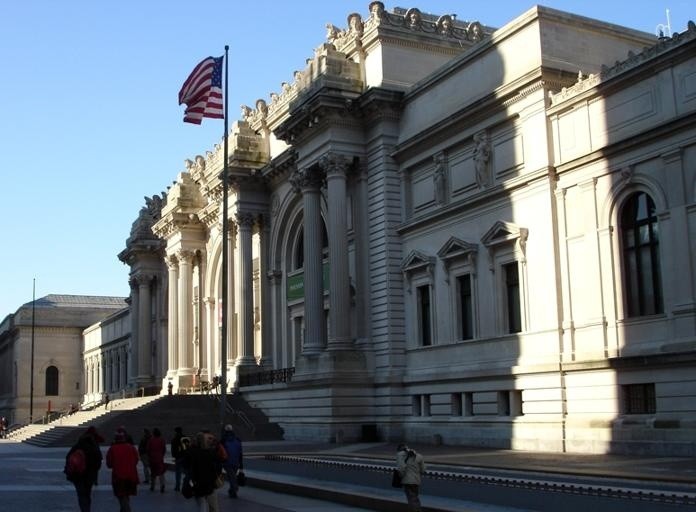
[142,480,165,494]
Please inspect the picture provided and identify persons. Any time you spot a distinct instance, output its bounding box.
[0,416,8,438]
[395,443,425,512]
[63,421,244,512]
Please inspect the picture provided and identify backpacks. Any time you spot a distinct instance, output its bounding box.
[180,436,191,451]
[64,448,88,492]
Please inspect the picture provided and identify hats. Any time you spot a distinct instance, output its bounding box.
[224,424,233,432]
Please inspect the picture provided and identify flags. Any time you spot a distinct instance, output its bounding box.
[177,54,225,125]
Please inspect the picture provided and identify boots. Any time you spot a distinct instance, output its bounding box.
[174,484,180,491]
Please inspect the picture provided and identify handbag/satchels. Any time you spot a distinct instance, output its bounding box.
[392,470,403,488]
[213,463,224,488]
[237,472,247,486]
